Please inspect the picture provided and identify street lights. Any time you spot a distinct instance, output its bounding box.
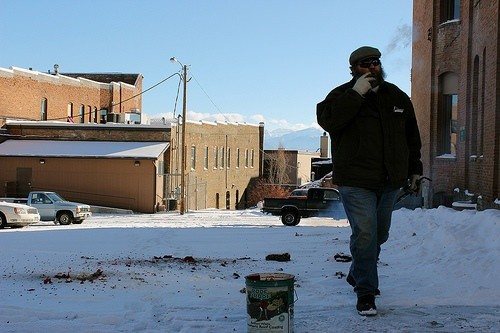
[170,56,188,214]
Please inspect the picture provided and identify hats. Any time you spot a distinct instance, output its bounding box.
[349,46,382,66]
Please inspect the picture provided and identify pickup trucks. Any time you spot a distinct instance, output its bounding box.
[0,191,92,226]
[261,187,347,227]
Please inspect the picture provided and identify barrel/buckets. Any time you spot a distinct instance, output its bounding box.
[244,271,298,333]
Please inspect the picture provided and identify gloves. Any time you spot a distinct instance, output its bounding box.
[351,71,376,98]
[410,174,423,198]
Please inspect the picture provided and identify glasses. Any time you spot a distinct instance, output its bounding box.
[355,59,380,68]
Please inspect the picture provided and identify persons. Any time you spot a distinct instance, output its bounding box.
[317,46,423,315]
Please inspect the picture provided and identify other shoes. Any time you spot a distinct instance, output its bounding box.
[356,295,378,316]
[346,275,382,298]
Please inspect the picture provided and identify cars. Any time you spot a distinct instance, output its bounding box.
[0,200,41,229]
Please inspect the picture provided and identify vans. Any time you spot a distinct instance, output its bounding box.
[287,189,310,199]
